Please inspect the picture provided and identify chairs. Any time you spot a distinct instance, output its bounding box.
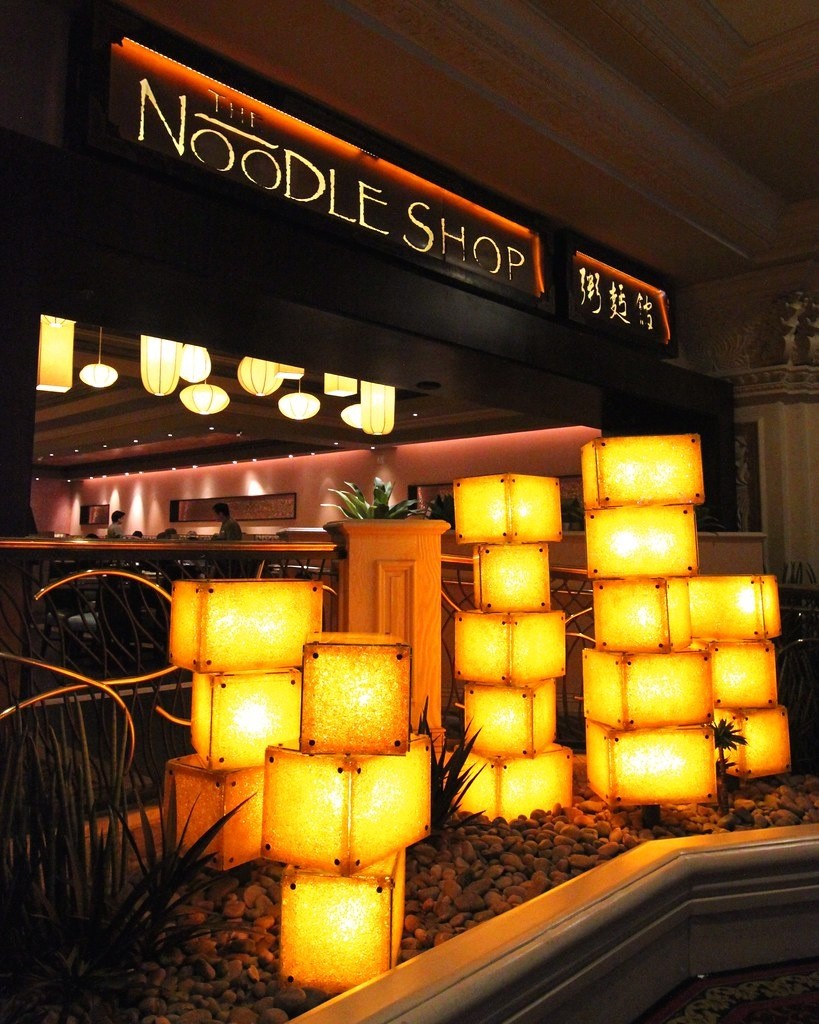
[32,554,293,688]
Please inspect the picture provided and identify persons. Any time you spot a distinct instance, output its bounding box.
[158,528,199,540]
[108,511,126,539]
[212,503,242,540]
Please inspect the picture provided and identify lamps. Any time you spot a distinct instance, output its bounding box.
[474,543,551,612]
[261,734,431,876]
[583,648,711,732]
[583,505,700,578]
[587,720,718,811]
[696,638,779,710]
[169,580,322,674]
[36,315,394,436]
[593,576,693,655]
[581,433,705,510]
[280,848,405,995]
[163,754,264,869]
[298,631,411,757]
[190,667,302,770]
[451,744,573,824]
[464,681,556,757]
[455,609,566,685]
[710,707,793,779]
[689,575,782,639]
[452,473,565,545]
